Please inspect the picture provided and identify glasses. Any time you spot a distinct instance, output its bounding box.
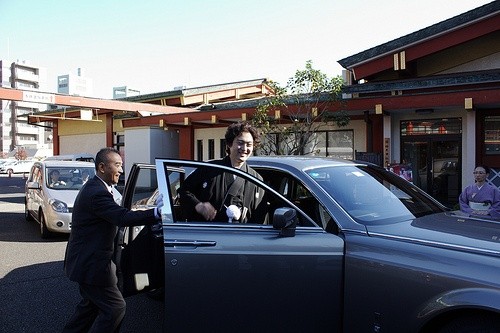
[473,171,486,175]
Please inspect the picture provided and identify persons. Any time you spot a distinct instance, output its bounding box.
[179,122,265,223]
[61,148,164,333]
[459,165,500,221]
[73,169,90,186]
[49,169,66,188]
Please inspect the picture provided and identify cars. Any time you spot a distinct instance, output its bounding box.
[114,151,500,333]
[24,159,124,240]
[42,154,96,164]
[0,158,40,175]
